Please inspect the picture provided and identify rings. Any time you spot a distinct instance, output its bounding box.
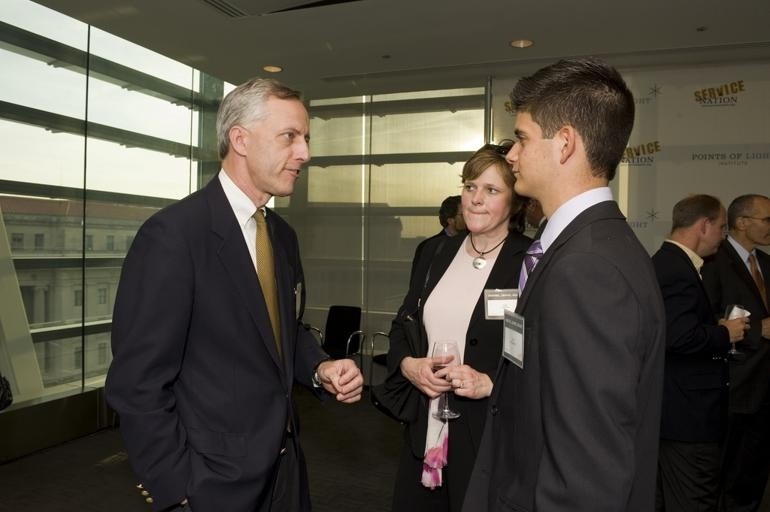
[460,379,464,389]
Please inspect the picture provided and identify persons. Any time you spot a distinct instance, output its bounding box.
[460,57,667,510]
[409,195,468,296]
[526,198,548,241]
[651,193,751,512]
[367,139,536,512]
[705,194,770,512]
[104,77,365,512]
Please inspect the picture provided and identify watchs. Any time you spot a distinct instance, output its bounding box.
[313,368,323,387]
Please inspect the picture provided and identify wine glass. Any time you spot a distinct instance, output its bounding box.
[432,341,461,418]
[726,304,744,355]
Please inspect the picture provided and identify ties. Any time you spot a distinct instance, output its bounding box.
[251,210,292,434]
[748,254,769,312]
[517,240,544,300]
[700,266,712,308]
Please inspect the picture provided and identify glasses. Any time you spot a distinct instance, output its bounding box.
[740,215,770,224]
[455,213,463,218]
[464,139,514,157]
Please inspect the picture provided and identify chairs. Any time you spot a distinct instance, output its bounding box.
[368,331,389,386]
[304,306,366,375]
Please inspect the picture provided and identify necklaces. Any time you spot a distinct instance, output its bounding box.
[470,231,508,270]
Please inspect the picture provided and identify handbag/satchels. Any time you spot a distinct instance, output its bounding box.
[370,237,448,424]
[0,374,13,411]
